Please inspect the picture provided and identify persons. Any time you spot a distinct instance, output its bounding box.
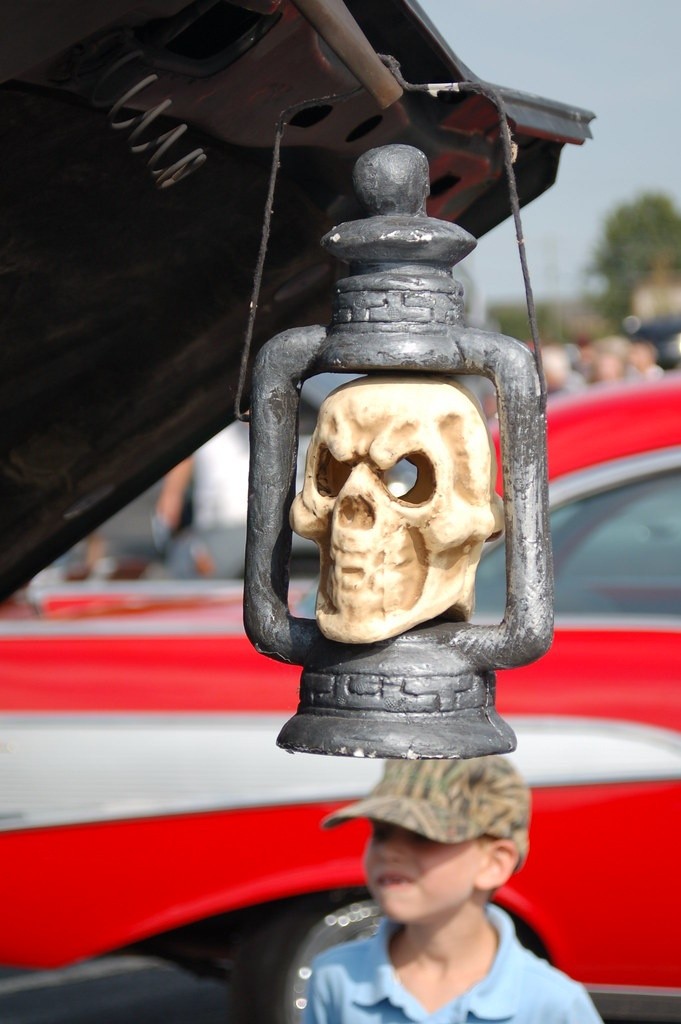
[539,334,667,399]
[140,421,251,579]
[295,752,604,1024]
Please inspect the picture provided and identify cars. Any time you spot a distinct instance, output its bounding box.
[0,384,680,1024]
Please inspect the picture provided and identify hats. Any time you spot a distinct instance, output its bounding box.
[322,752,531,875]
